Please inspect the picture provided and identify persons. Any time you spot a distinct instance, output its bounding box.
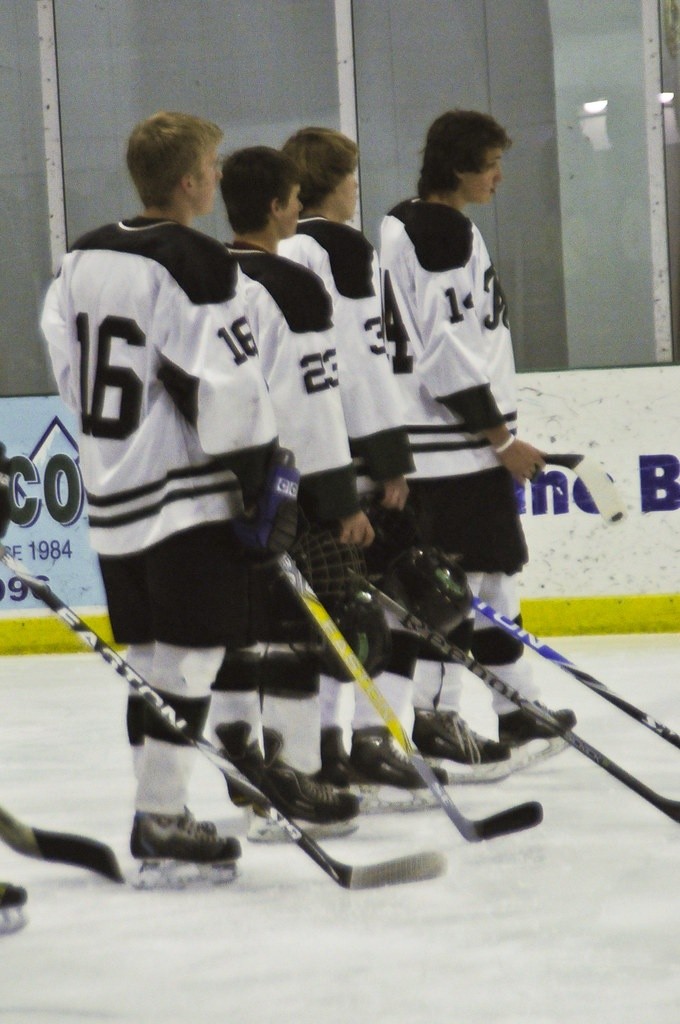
[276,124,448,813]
[201,144,376,838]
[376,109,576,782]
[36,110,296,891]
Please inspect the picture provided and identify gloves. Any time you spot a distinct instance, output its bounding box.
[242,449,301,564]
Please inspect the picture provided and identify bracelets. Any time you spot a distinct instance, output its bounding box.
[492,433,515,453]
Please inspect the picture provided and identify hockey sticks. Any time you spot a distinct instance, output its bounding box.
[543,453,629,527]
[277,549,544,844]
[469,595,680,753]
[346,567,680,823]
[0,543,448,891]
[1,809,123,883]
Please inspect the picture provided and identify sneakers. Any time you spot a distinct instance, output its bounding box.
[130,806,240,892]
[347,726,450,813]
[499,702,577,774]
[0,881,27,935]
[318,725,354,795]
[249,729,360,844]
[216,719,262,807]
[412,708,516,783]
